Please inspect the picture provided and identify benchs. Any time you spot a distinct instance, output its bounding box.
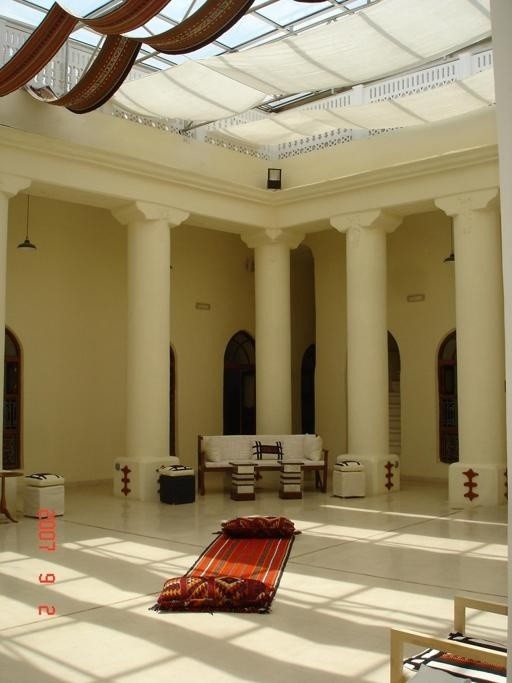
[197,434,330,496]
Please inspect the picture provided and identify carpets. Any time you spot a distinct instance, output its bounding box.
[157,535,294,612]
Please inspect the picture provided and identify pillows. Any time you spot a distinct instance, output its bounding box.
[23,472,65,486]
[334,460,363,471]
[157,576,270,611]
[158,464,194,476]
[221,516,295,537]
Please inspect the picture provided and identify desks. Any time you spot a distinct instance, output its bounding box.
[0,471,24,523]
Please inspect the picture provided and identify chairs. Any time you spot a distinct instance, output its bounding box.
[389,594,512,682]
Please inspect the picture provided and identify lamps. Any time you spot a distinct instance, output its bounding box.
[17,194,37,253]
[442,217,456,264]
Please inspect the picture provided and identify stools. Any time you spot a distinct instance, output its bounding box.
[332,471,367,498]
[23,484,66,520]
[159,476,195,507]
